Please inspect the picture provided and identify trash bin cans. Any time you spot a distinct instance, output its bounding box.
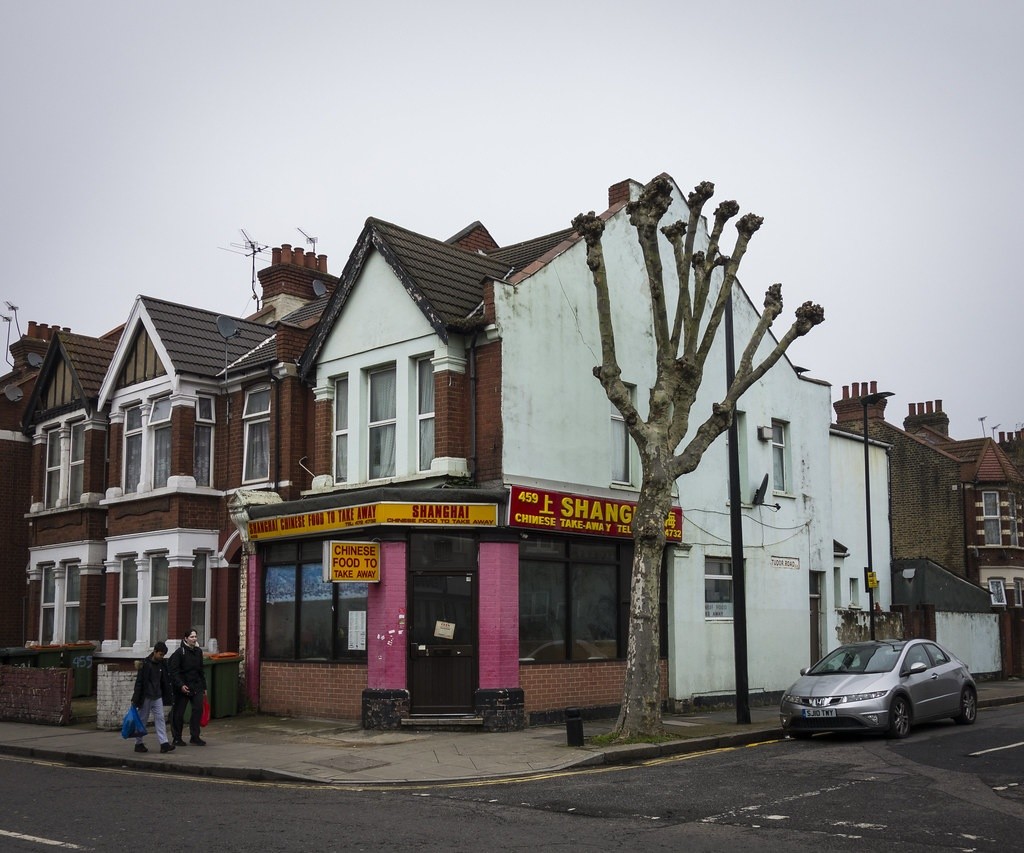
[183,655,213,723]
[0,647,36,668]
[64,645,96,698]
[213,651,243,718]
[34,648,63,668]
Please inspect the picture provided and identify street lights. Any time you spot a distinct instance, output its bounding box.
[710,252,754,724]
[861,390,900,642]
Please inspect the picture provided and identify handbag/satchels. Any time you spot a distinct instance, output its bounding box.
[200,695,210,726]
[122,704,148,739]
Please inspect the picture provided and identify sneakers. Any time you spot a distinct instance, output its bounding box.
[134,744,148,752]
[160,743,175,753]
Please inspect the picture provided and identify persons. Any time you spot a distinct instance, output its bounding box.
[169,629,207,747]
[130,642,175,753]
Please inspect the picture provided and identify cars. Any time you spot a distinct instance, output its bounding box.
[778,639,979,738]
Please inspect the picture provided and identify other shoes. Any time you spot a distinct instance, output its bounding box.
[173,738,186,746]
[191,737,206,745]
